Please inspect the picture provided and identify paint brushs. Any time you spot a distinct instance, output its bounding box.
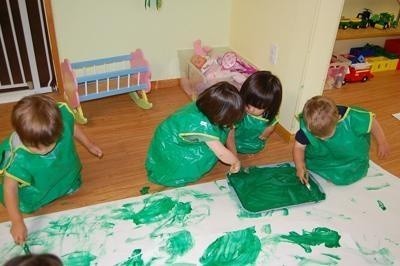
[23,243,32,256]
[303,177,313,192]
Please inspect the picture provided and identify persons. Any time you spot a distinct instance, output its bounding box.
[226,69,283,159]
[0,92,103,247]
[143,80,244,188]
[292,95,390,187]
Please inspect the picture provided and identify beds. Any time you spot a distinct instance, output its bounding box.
[62,49,154,123]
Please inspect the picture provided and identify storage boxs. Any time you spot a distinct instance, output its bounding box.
[323,36,400,91]
[176,45,259,101]
